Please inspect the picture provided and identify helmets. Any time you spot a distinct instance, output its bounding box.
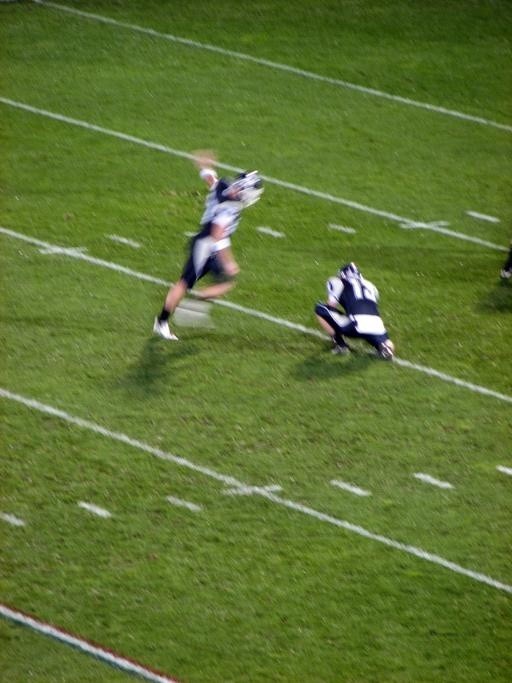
[340,261,363,278]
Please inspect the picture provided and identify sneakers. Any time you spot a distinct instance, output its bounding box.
[331,344,351,356]
[379,343,393,361]
[153,315,178,341]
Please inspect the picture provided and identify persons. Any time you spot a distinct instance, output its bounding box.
[152,145,267,341]
[314,260,395,359]
[500,250,511,278]
